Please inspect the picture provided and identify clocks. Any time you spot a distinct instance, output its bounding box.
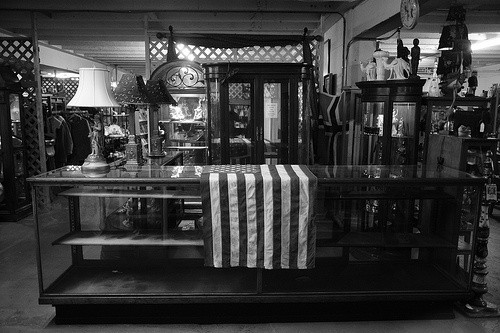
[399,0,420,30]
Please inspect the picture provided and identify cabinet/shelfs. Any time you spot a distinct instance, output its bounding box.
[207,63,308,164]
[346,79,426,240]
[29,160,485,327]
[428,97,500,271]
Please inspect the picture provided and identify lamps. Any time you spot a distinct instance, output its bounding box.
[66,68,178,176]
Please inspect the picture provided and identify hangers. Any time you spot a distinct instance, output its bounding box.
[46,112,89,123]
